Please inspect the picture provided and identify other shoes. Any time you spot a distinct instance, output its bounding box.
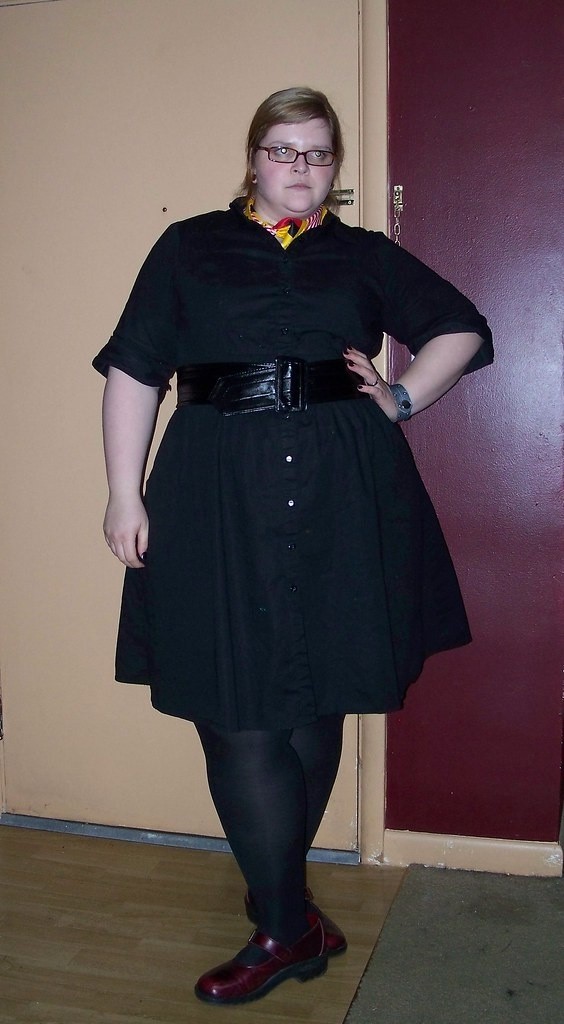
[194,911,329,1005]
[304,887,348,955]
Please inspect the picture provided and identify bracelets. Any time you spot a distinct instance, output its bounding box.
[389,384,413,421]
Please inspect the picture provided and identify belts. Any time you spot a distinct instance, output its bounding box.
[176,355,370,417]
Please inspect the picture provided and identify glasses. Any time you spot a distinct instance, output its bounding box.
[256,146,336,166]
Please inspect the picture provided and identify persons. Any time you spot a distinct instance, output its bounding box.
[91,88,494,1005]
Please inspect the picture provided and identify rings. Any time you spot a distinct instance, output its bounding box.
[365,378,380,386]
